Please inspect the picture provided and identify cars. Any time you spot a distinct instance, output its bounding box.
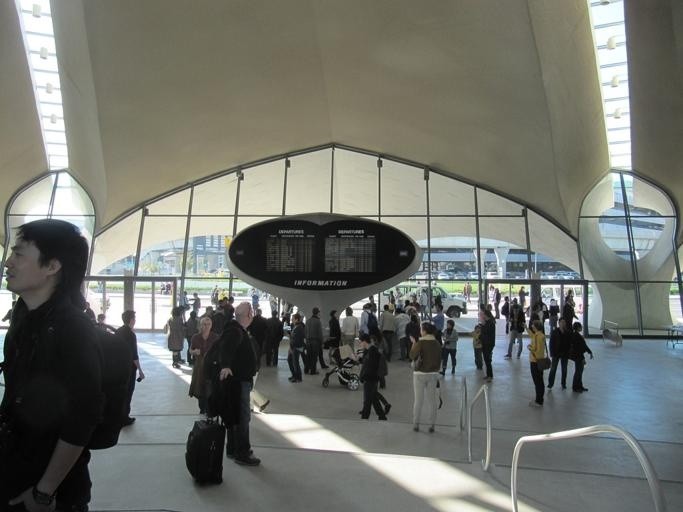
[406,271,579,282]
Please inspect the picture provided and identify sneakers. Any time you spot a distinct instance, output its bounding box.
[548,383,553,388]
[439,372,444,375]
[359,404,391,420]
[260,400,270,411]
[529,401,542,407]
[483,376,488,379]
[227,450,261,466]
[288,366,329,382]
[562,384,566,388]
[575,386,588,393]
[505,355,520,359]
[122,418,135,427]
[487,377,492,383]
[452,370,455,373]
[413,427,434,433]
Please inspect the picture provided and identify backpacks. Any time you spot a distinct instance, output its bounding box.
[85,321,134,451]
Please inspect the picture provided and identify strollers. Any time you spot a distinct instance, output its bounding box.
[321,337,363,391]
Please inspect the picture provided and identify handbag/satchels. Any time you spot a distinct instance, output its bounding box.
[566,349,582,362]
[411,355,423,371]
[441,348,448,360]
[199,383,226,417]
[378,354,387,377]
[538,358,551,369]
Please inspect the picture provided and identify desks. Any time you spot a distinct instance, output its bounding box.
[664,323,683,349]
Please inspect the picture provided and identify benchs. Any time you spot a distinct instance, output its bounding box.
[602,319,622,347]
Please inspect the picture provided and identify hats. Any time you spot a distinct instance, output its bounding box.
[533,320,543,330]
[313,308,320,312]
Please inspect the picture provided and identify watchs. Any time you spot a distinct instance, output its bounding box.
[31,486,58,506]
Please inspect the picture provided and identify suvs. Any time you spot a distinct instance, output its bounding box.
[378,283,466,318]
[523,285,592,317]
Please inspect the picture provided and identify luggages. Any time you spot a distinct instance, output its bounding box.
[185,380,226,488]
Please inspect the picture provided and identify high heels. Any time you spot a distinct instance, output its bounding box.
[178,359,185,364]
[172,363,181,368]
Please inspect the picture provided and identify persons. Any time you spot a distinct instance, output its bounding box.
[489,285,530,359]
[213,302,261,466]
[523,317,593,406]
[188,317,221,423]
[360,281,458,375]
[114,310,145,424]
[85,301,96,322]
[247,331,270,413]
[357,334,391,420]
[96,313,109,333]
[530,290,579,333]
[409,322,442,432]
[472,304,495,382]
[286,308,330,383]
[1,300,17,325]
[161,282,234,369]
[0,218,107,511]
[249,288,294,367]
[463,281,472,303]
[329,307,359,363]
